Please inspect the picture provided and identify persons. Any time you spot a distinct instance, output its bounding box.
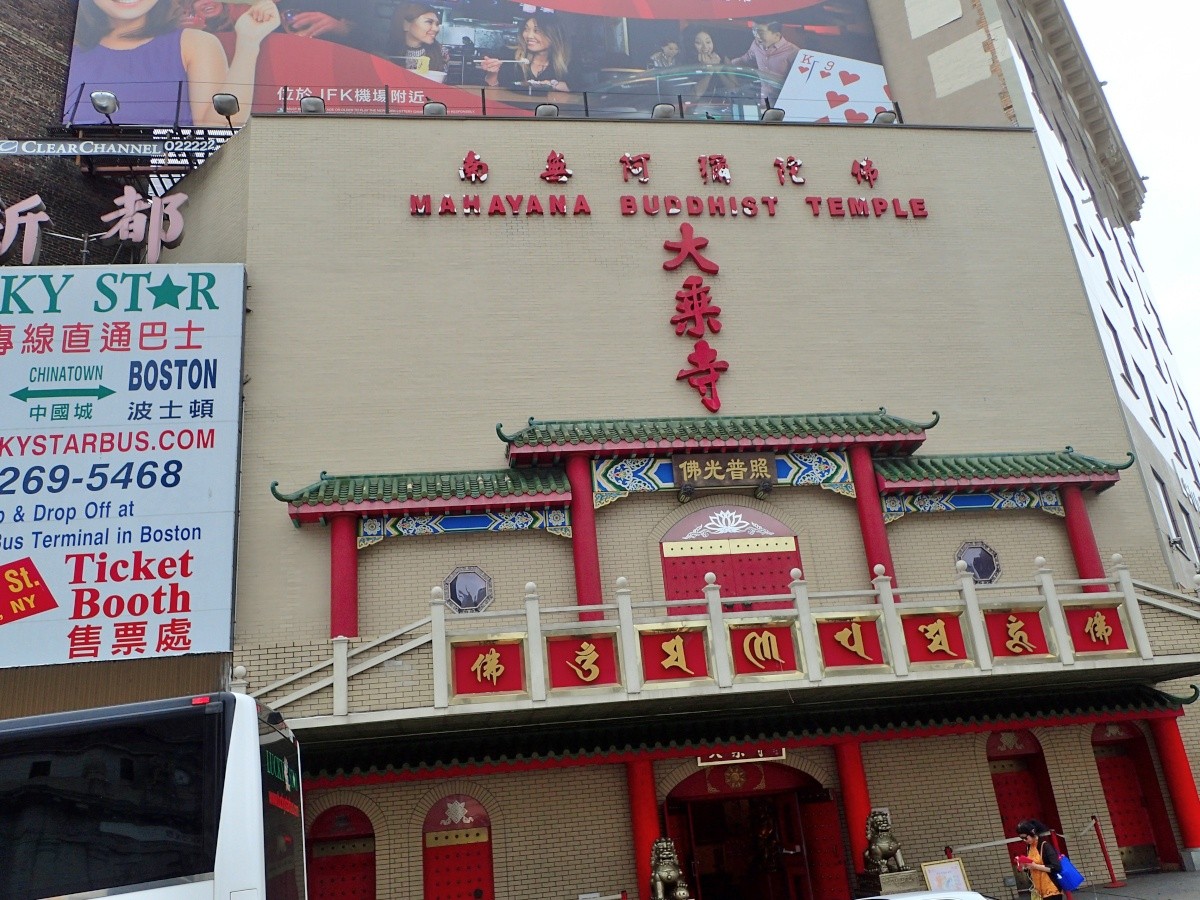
[64,0,802,145]
[1014,818,1063,900]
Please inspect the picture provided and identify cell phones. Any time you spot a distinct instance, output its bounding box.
[1016,855,1034,864]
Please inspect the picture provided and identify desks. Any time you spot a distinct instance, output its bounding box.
[451,84,549,103]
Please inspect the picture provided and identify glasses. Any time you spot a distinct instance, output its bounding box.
[1021,835,1028,840]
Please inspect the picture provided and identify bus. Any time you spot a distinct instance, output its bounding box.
[1,691,309,900]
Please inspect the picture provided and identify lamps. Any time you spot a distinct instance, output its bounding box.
[651,103,675,119]
[212,93,240,135]
[535,104,560,118]
[422,102,448,116]
[89,90,124,126]
[872,111,896,124]
[761,108,785,121]
[300,96,326,114]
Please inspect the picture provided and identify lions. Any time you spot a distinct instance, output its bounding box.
[650,838,689,900]
[864,810,912,876]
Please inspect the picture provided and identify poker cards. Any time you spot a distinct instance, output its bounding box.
[772,48,898,124]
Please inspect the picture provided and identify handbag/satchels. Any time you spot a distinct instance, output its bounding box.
[1041,841,1084,891]
